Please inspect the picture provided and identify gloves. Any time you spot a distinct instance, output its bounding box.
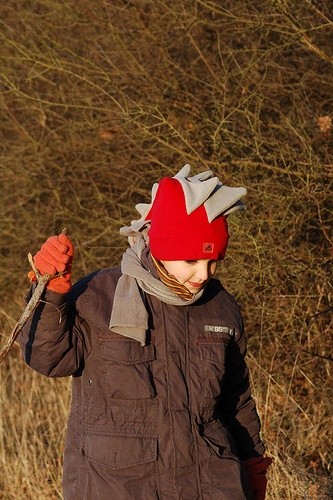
[26,233,73,293]
[239,456,272,500]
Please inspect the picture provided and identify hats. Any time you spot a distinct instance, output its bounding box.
[132,164,247,261]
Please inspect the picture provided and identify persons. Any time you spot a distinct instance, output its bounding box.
[12,162,273,500]
